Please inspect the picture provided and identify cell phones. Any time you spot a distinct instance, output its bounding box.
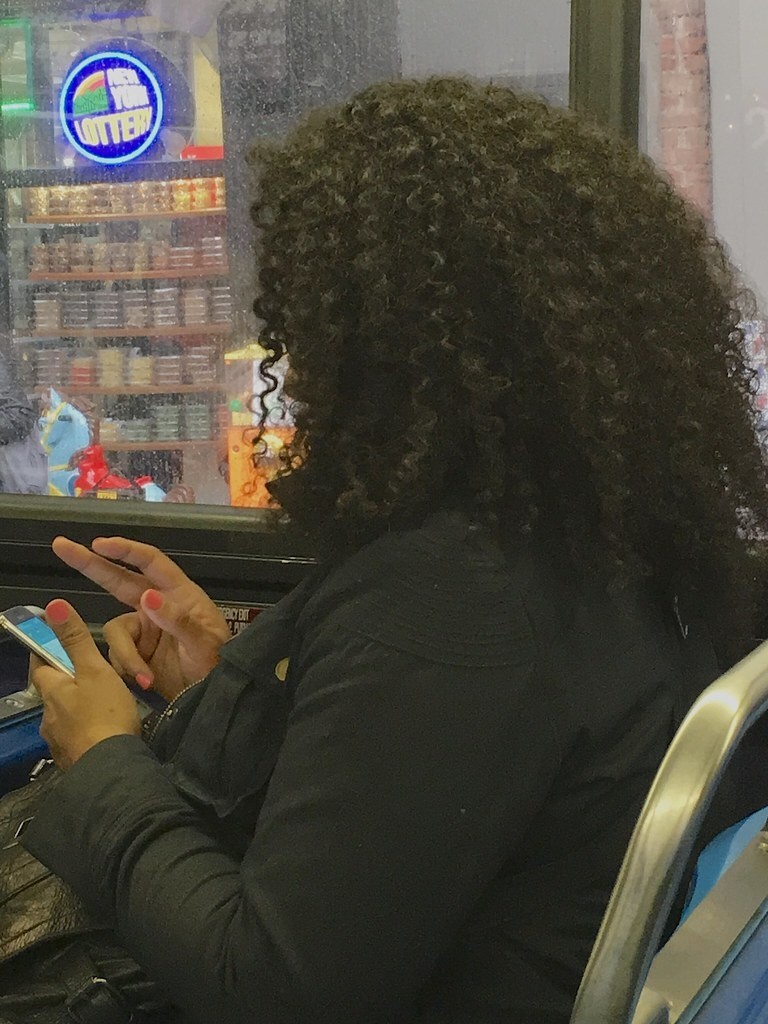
[0,606,154,727]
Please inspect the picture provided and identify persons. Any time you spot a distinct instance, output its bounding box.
[0,352,51,495]
[0,71,768,1024]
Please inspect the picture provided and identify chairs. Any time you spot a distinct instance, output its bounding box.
[557,629,765,1024]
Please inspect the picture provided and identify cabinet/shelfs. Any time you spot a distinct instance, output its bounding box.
[4,158,235,454]
